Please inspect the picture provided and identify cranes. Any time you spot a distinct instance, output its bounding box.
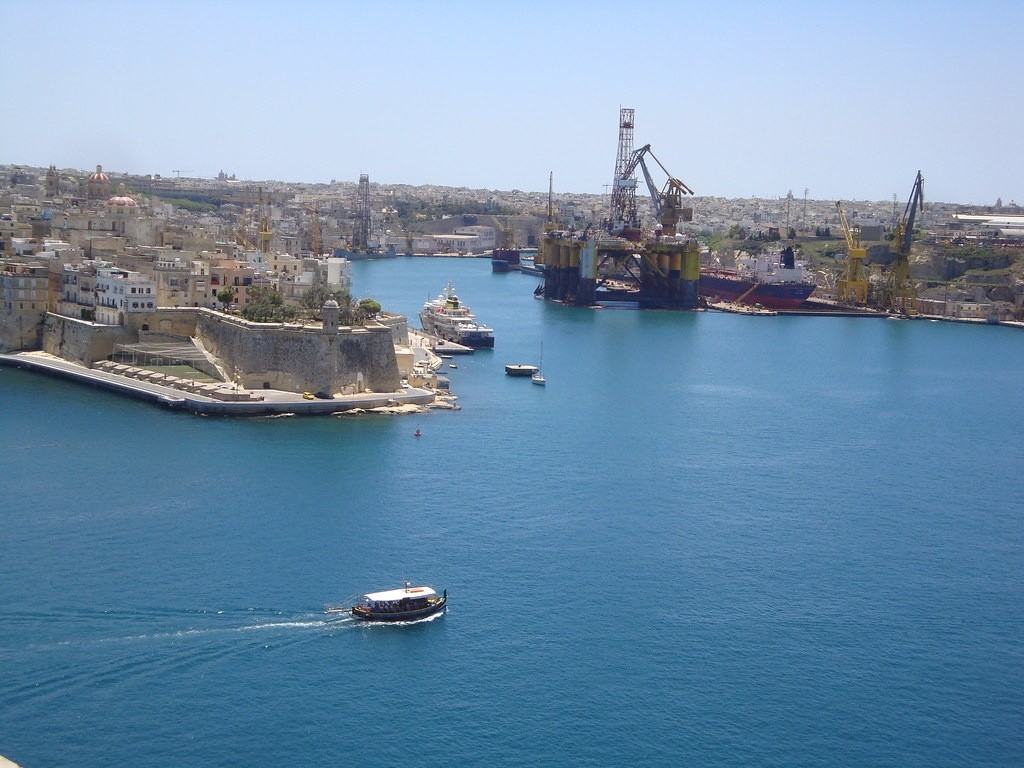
[618,144,695,235]
[878,169,926,316]
[834,200,869,306]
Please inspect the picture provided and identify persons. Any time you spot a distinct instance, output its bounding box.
[354,599,432,612]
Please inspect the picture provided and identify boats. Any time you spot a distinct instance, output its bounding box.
[348,581,448,624]
[532,341,546,386]
[418,280,495,351]
